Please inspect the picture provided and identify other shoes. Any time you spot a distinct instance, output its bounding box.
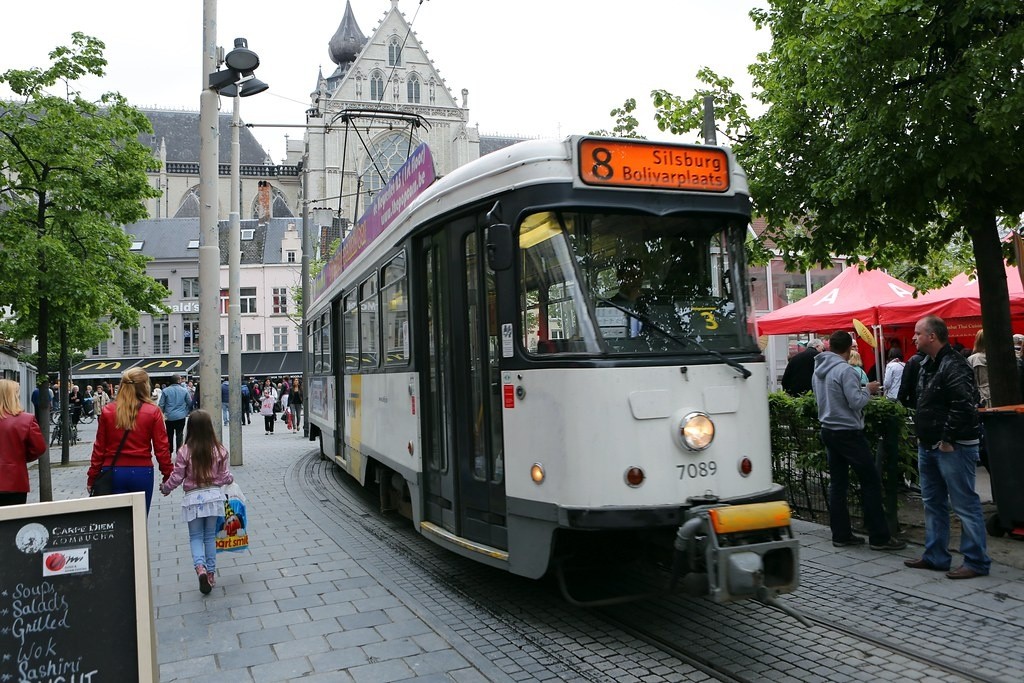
[264,433,273,435]
[196,564,216,595]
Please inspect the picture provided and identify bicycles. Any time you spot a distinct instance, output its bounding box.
[49,403,95,448]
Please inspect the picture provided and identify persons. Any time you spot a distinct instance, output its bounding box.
[86,366,173,518]
[28,376,303,434]
[0,378,46,505]
[598,257,668,310]
[159,410,233,595]
[156,373,191,453]
[780,329,1023,578]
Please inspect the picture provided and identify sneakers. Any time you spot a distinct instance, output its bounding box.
[870,536,907,549]
[831,532,866,547]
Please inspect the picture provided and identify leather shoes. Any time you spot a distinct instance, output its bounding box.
[946,565,980,578]
[904,557,949,570]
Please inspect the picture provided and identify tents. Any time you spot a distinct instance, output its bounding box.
[877,231,1024,396]
[745,257,923,397]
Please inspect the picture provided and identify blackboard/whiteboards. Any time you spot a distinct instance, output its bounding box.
[0,491,160,683]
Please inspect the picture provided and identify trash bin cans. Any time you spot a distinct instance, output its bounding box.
[977,404,1024,541]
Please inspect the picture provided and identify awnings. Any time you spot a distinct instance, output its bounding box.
[313,349,408,372]
[57,354,199,379]
[192,349,302,378]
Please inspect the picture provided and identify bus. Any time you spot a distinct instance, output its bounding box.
[302,133,814,630]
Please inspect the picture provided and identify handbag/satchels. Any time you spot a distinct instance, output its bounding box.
[260,397,274,416]
[281,408,293,430]
[215,481,248,554]
[89,467,115,498]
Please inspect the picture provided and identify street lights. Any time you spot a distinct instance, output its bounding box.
[200,71,236,453]
[228,40,269,464]
